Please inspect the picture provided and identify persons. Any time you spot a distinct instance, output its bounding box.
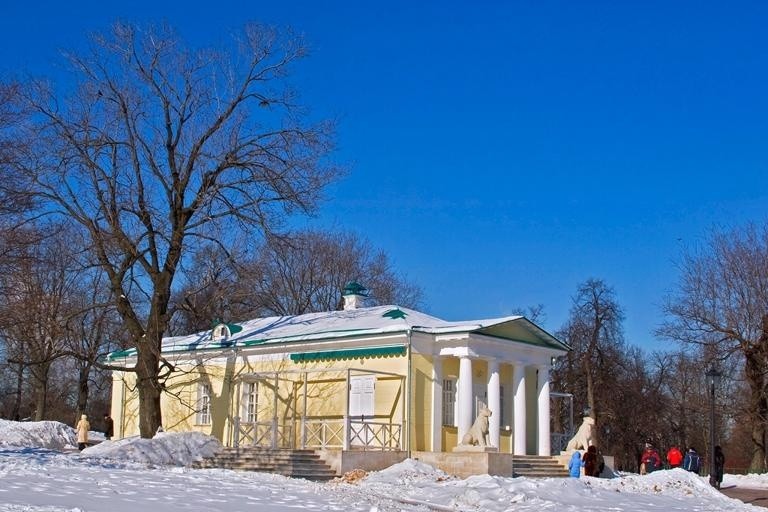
[641,446,660,472]
[75,414,90,450]
[102,413,114,439]
[569,451,586,477]
[709,445,725,490]
[667,443,683,469]
[582,445,599,477]
[597,450,605,478]
[683,447,703,474]
[641,439,659,457]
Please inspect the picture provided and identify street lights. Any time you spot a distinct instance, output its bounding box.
[705,366,720,487]
[603,423,610,456]
